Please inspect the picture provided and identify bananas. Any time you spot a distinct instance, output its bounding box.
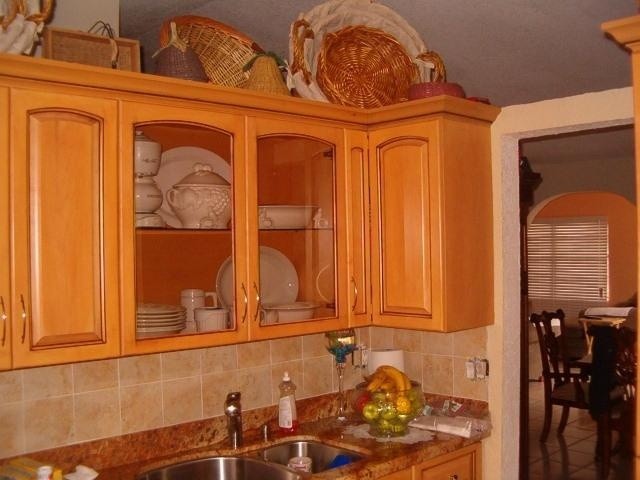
[366,366,412,398]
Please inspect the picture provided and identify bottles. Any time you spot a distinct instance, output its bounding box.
[278,371,300,435]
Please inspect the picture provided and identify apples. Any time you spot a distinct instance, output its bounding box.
[355,393,397,433]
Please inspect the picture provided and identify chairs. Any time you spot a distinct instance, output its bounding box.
[530,296,636,477]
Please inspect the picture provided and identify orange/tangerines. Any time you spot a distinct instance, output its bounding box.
[395,396,412,415]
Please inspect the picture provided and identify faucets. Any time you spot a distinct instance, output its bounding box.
[224,391,243,443]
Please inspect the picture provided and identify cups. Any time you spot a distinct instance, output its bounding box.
[180,289,218,332]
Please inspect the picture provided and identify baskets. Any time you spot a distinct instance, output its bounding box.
[288,0,446,110]
[159,15,298,98]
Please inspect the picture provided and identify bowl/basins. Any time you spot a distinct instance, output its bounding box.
[258,205,319,229]
[262,302,322,324]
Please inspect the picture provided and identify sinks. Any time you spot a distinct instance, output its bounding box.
[252,437,369,480]
[132,454,301,480]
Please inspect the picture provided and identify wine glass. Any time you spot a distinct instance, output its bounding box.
[350,378,426,439]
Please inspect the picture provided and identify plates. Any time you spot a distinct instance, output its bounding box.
[216,244,300,323]
[135,302,187,339]
[152,146,231,229]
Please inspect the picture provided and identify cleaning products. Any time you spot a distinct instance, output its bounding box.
[278,370,297,434]
